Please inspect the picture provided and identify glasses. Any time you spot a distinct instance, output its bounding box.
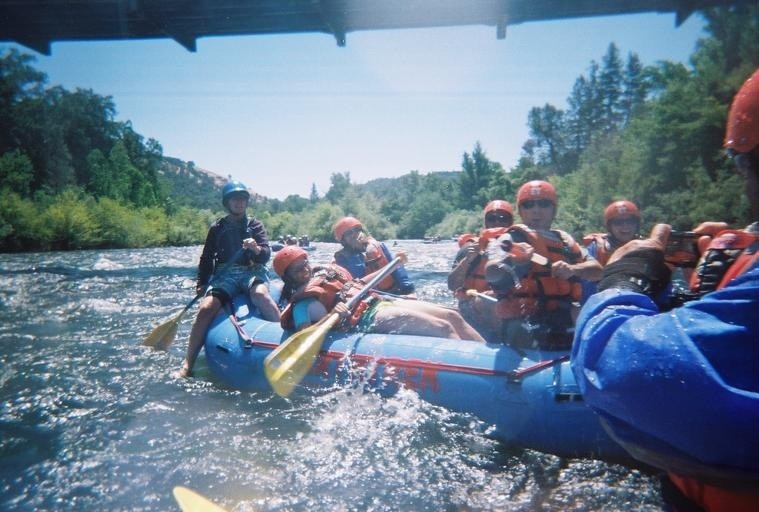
[486,212,512,223]
[343,226,362,237]
[292,259,308,272]
[521,200,552,209]
[610,218,638,226]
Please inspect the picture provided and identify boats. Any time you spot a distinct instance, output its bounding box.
[271,239,316,252]
[391,242,400,248]
[202,280,633,462]
[419,236,438,244]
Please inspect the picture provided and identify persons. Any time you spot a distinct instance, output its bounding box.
[328,216,418,298]
[272,244,489,343]
[431,233,441,244]
[167,181,285,381]
[275,234,316,248]
[392,240,398,247]
[578,201,642,314]
[445,198,520,347]
[570,70,758,512]
[483,178,605,350]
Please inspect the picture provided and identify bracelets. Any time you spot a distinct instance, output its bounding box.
[456,262,467,273]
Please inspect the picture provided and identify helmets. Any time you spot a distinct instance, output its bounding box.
[334,216,362,241]
[722,68,758,154]
[221,180,250,207]
[517,180,557,219]
[273,244,308,278]
[484,200,514,223]
[605,201,640,232]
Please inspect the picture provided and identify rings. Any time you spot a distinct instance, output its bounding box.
[556,266,563,273]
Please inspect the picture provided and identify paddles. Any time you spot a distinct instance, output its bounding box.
[172,485,223,512]
[263,257,403,398]
[143,244,249,352]
